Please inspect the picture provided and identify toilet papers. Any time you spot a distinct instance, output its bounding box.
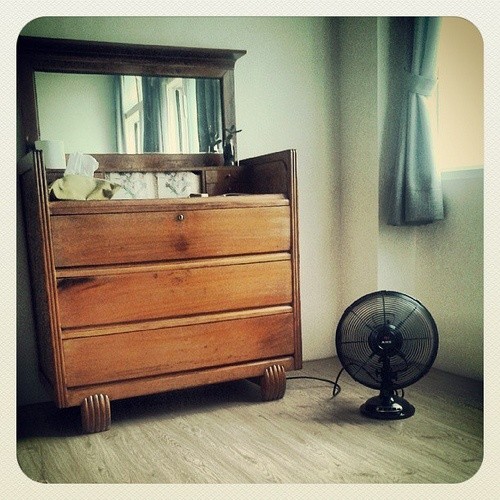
[42,139,67,169]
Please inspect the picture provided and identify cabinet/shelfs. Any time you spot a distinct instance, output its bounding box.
[18,149,303,433]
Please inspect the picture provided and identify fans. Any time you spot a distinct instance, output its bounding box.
[335,290,439,421]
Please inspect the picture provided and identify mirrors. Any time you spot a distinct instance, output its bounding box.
[17,35,248,170]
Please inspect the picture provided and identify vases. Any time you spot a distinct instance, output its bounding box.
[223,139,235,166]
